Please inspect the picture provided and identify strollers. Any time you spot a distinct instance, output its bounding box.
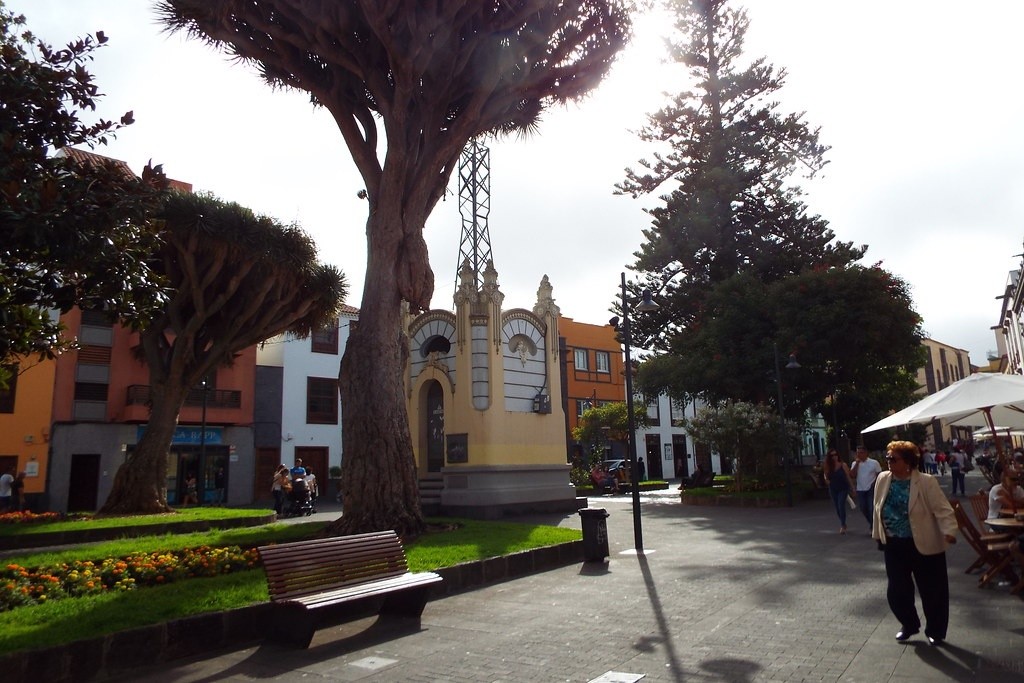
[281,479,314,518]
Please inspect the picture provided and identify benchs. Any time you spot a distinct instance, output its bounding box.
[256,530,442,651]
[588,475,630,495]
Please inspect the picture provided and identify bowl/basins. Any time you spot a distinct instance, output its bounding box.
[1015,514,1024,522]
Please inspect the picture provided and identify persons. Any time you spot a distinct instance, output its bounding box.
[603,465,619,492]
[681,451,688,476]
[675,456,682,477]
[823,448,857,534]
[290,457,306,487]
[872,440,958,645]
[813,461,825,487]
[722,456,732,475]
[637,456,645,482]
[302,466,316,500]
[271,465,290,514]
[647,450,662,477]
[946,434,975,463]
[919,447,968,497]
[982,443,1024,541]
[849,445,882,536]
[0,465,16,513]
[591,464,619,496]
[182,470,198,508]
[12,470,26,512]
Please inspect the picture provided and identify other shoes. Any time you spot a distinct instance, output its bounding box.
[928,634,943,644]
[615,488,620,491]
[895,628,920,640]
[840,523,847,534]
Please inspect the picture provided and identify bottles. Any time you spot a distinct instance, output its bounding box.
[1011,461,1014,470]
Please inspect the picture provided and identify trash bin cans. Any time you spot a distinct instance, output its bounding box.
[578,508,610,562]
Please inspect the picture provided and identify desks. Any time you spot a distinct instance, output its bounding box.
[980,518,1024,588]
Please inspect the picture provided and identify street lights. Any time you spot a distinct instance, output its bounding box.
[621,272,660,551]
[773,342,801,507]
[584,388,599,451]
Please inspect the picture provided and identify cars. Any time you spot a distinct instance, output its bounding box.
[590,459,632,479]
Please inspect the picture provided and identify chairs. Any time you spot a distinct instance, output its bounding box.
[951,465,1024,594]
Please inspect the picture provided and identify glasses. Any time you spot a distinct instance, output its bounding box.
[886,455,903,465]
[831,454,838,457]
[1007,477,1020,482]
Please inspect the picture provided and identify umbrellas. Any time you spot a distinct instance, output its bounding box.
[984,430,1024,436]
[974,434,986,439]
[973,426,1012,434]
[860,371,1024,518]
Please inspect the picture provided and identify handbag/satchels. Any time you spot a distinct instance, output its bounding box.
[961,452,974,472]
